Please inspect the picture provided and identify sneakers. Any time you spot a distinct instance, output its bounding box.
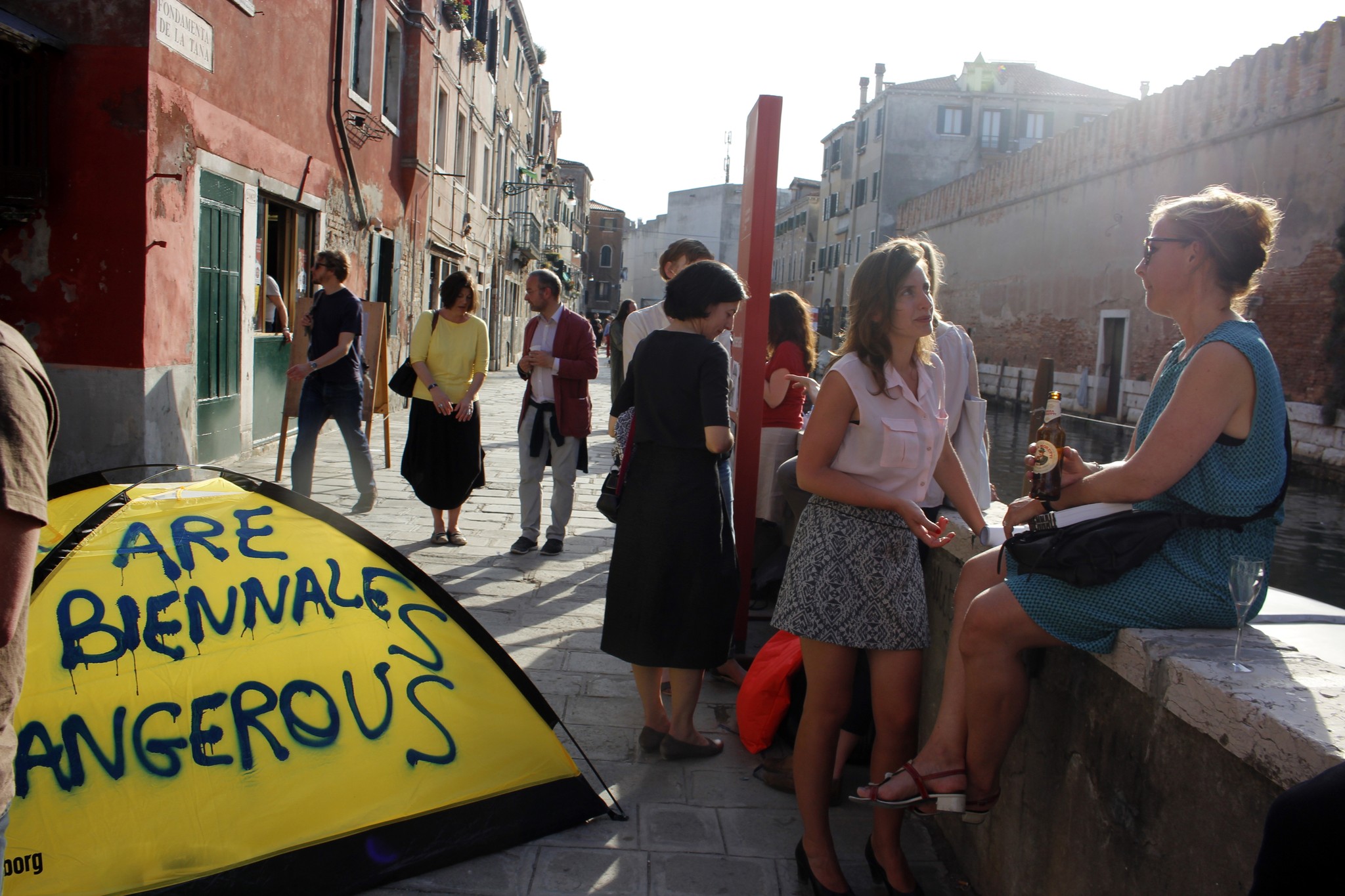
[510,537,538,554]
[351,488,378,512]
[540,539,563,554]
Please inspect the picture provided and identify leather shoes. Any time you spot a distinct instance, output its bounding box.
[638,726,667,753]
[660,734,724,760]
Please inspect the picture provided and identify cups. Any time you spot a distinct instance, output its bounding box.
[529,345,541,359]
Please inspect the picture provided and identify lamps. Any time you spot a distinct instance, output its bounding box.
[567,273,594,281]
[503,181,576,207]
[545,244,581,258]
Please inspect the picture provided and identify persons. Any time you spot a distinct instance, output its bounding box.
[751,290,814,594]
[599,237,737,761]
[400,271,490,545]
[768,237,999,896]
[577,312,615,358]
[510,269,599,555]
[0,317,62,895]
[287,249,378,514]
[258,270,292,344]
[845,187,1295,826]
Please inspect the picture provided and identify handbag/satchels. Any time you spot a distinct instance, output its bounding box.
[596,453,622,522]
[388,356,418,399]
[996,511,1202,586]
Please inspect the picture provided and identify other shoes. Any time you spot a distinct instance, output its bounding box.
[711,672,740,687]
[660,682,672,695]
[753,765,842,806]
[446,530,467,545]
[432,531,448,544]
[748,596,776,621]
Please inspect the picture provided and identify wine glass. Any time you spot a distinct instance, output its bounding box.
[1216,555,1265,674]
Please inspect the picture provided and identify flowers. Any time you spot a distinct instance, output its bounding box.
[562,279,575,289]
[445,0,471,24]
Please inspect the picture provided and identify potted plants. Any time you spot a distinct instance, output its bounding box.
[467,37,486,62]
[545,252,559,261]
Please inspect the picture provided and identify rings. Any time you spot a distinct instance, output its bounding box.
[1003,520,1007,527]
[466,409,471,415]
[438,404,443,408]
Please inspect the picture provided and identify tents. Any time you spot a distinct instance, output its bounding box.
[1,460,627,896]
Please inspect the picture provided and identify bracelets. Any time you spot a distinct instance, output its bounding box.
[282,325,290,331]
[1089,461,1103,471]
[1039,500,1058,512]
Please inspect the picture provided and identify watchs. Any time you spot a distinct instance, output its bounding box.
[309,361,317,371]
[428,383,438,392]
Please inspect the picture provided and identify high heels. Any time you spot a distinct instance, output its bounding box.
[848,759,967,814]
[796,837,852,895]
[865,835,922,896]
[911,792,1001,824]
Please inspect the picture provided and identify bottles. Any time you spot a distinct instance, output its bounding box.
[1031,391,1065,501]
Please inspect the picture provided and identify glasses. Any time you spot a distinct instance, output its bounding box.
[1144,236,1193,264]
[315,263,330,269]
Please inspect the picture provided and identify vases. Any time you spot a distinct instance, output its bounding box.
[444,6,465,29]
[565,286,572,291]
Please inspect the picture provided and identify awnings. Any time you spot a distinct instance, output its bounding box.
[519,167,539,180]
